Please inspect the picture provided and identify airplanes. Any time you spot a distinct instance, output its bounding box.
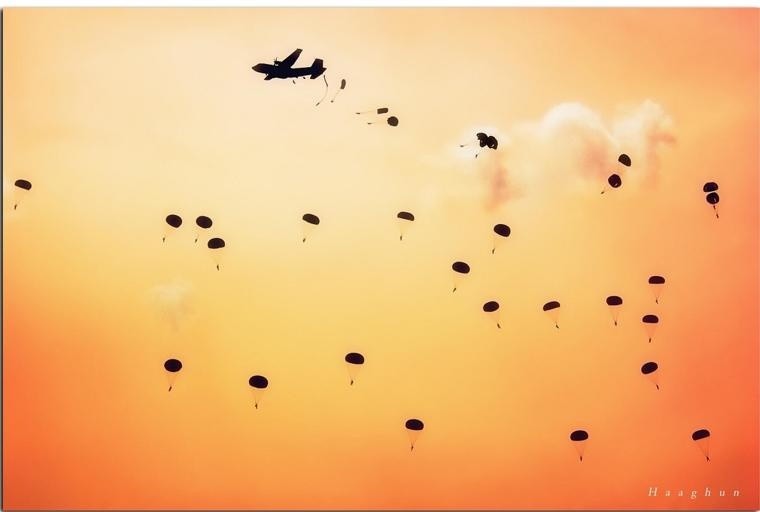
[252,49,326,83]
